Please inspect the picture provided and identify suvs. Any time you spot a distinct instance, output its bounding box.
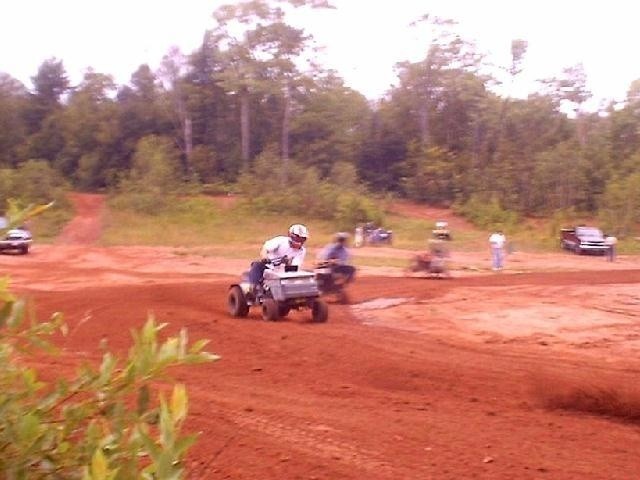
[431,220,452,242]
[358,228,392,246]
[226,253,331,325]
[303,260,357,302]
[0,213,34,256]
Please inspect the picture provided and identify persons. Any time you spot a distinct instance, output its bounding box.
[602,233,618,262]
[317,233,358,295]
[432,221,453,255]
[354,224,364,249]
[249,223,309,303]
[488,229,507,273]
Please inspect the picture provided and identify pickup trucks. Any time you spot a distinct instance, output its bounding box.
[559,225,613,257]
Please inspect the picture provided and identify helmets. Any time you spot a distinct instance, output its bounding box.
[287,223,309,241]
